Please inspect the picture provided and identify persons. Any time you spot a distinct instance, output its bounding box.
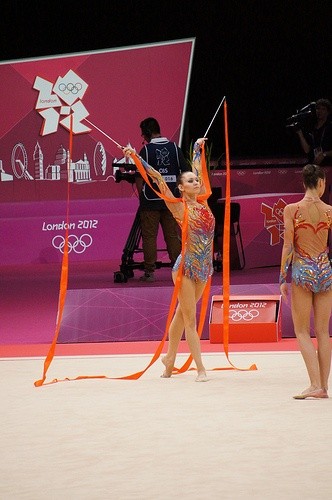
[136,118,189,281]
[120,137,215,382]
[279,164,332,399]
[295,99,332,167]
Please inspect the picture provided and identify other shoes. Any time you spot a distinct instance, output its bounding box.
[140,273,154,281]
